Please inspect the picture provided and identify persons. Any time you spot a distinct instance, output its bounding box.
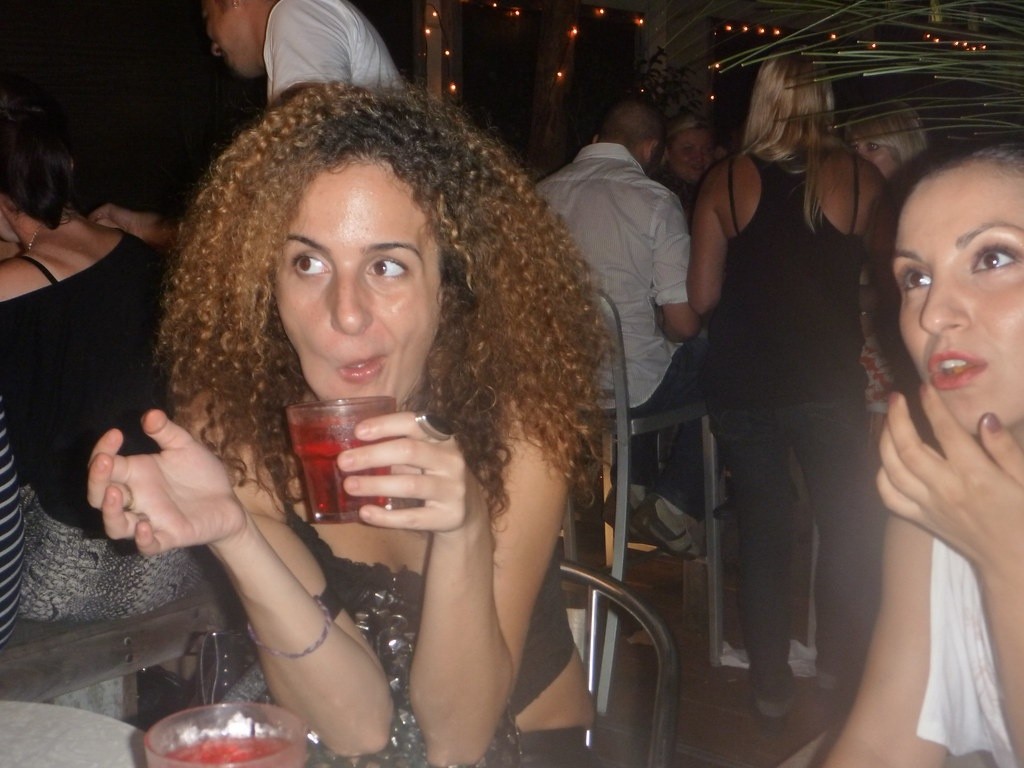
[0,0,1024,768]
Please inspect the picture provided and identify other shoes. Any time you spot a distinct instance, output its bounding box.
[749,689,785,720]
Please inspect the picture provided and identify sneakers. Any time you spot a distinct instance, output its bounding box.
[631,495,701,559]
[604,489,658,546]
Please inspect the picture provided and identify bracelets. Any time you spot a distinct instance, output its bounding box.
[246,601,331,658]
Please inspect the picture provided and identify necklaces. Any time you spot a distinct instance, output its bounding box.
[27,224,44,250]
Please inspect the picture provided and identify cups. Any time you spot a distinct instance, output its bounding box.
[146,703,309,766]
[288,397,397,523]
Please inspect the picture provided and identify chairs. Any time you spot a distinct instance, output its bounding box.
[1,289,850,767]
